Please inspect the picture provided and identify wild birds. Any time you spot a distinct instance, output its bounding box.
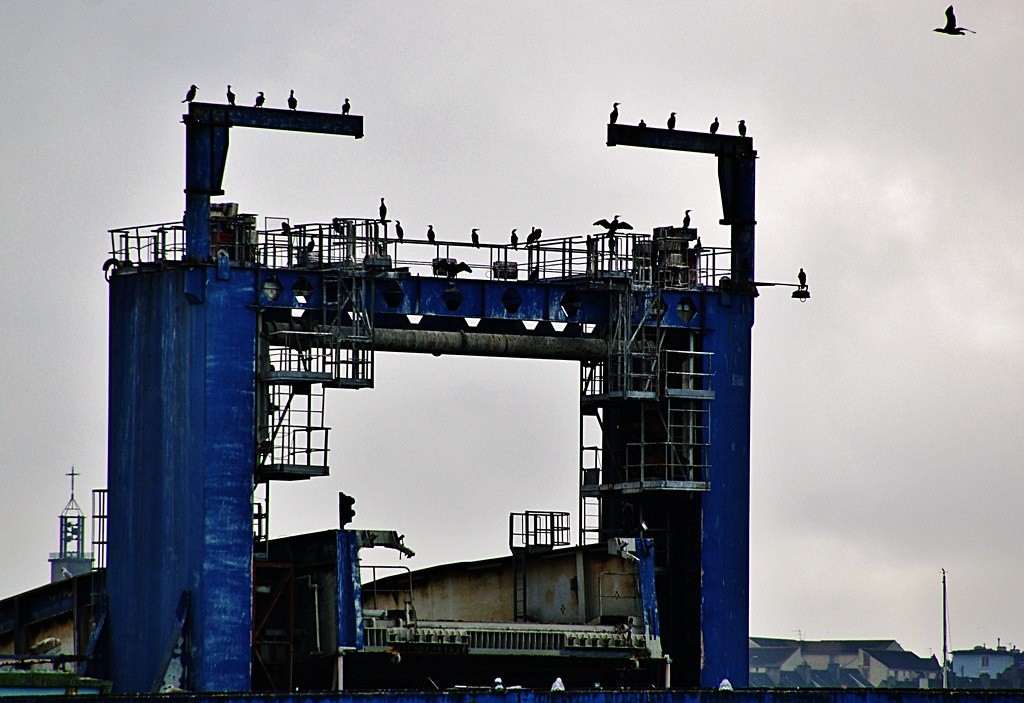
[609,102,747,136]
[798,268,806,285]
[593,215,633,236]
[181,84,351,116]
[682,210,693,228]
[394,219,542,252]
[307,235,315,252]
[694,236,701,255]
[379,197,387,220]
[933,5,977,36]
